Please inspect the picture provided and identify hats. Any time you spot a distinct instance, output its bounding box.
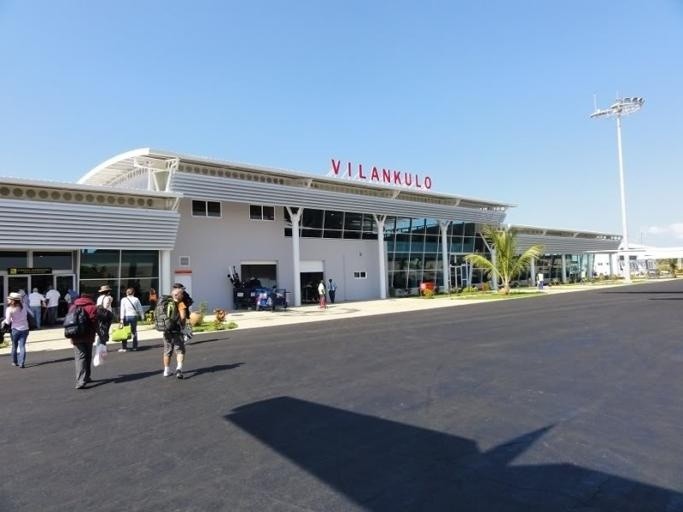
[98,285,113,292]
[173,283,184,288]
[7,292,22,301]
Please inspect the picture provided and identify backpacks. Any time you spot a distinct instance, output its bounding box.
[98,309,112,320]
[63,310,88,341]
[154,298,179,331]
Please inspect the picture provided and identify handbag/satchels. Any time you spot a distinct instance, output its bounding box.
[113,326,132,341]
[136,315,141,321]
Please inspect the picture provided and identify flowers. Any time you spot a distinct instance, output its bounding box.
[214,309,227,322]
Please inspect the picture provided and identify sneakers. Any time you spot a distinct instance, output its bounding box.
[11,362,17,367]
[76,381,86,391]
[131,348,138,351]
[118,348,127,353]
[162,370,173,377]
[176,370,184,380]
[20,363,25,368]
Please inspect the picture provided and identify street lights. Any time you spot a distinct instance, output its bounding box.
[590,94,646,285]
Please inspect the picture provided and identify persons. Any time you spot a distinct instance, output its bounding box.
[328,279,336,302]
[537,267,544,289]
[317,280,326,309]
[4,282,193,388]
